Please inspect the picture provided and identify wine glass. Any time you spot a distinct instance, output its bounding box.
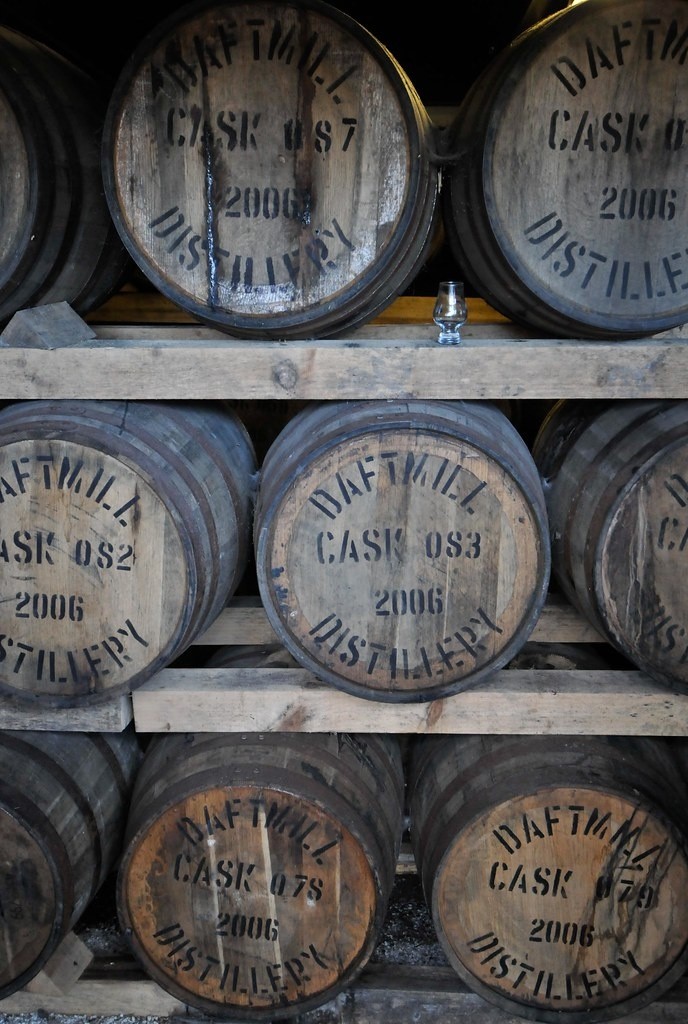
[432,281,469,344]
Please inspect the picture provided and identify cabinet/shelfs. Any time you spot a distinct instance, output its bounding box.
[1,295,687,1024]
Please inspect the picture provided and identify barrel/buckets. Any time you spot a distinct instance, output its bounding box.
[100,1,447,341]
[115,733,406,1024]
[0,26,124,328]
[400,735,688,1024]
[533,399,688,696]
[0,730,142,1002]
[0,399,252,710]
[254,398,551,705]
[449,0,688,340]
